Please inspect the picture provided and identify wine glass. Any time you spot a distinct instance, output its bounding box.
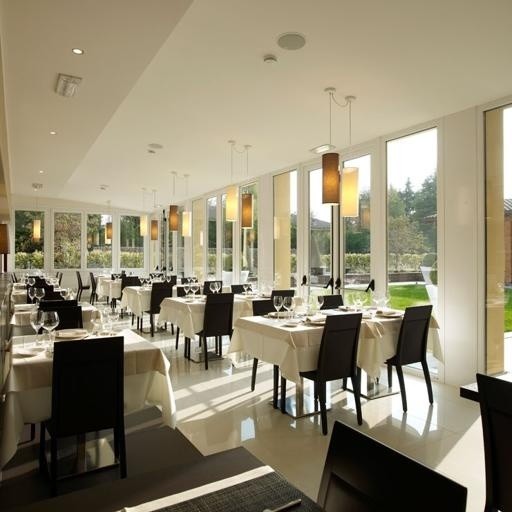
[24,276,120,348]
[136,271,392,319]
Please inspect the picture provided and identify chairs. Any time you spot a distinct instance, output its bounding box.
[40,336,127,498]
[251,300,284,409]
[317,420,468,511]
[317,295,347,391]
[7,270,295,336]
[184,293,234,369]
[280,313,363,435]
[476,373,512,512]
[386,305,433,413]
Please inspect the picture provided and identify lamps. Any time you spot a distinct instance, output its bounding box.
[140,171,192,240]
[32,183,43,243]
[241,145,254,229]
[226,141,240,221]
[88,215,112,247]
[322,88,340,206]
[340,96,359,217]
